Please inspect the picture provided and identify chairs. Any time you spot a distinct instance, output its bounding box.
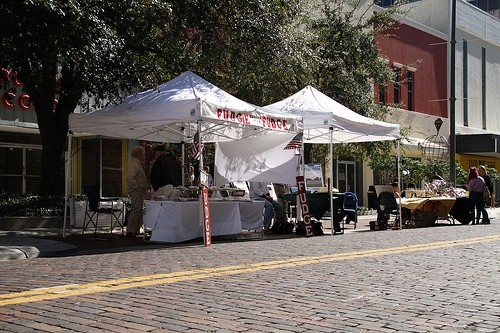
[81,184,124,241]
[378,191,404,227]
[344,192,358,230]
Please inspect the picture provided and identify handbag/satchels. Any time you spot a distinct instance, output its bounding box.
[271,219,294,234]
[296,220,324,236]
[483,187,490,201]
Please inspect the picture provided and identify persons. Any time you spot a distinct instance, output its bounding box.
[149,146,176,191]
[126,147,147,240]
[391,165,493,224]
[249,182,284,231]
[273,183,292,225]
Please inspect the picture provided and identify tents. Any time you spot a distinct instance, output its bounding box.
[62,71,304,247]
[257,84,403,230]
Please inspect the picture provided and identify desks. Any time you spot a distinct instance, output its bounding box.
[280,193,345,235]
[396,197,456,224]
[143,200,265,243]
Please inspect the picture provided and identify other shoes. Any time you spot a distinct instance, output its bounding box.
[127,232,144,239]
[263,230,273,233]
[480,219,489,224]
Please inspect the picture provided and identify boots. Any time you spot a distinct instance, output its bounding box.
[471,212,475,225]
[476,212,480,224]
